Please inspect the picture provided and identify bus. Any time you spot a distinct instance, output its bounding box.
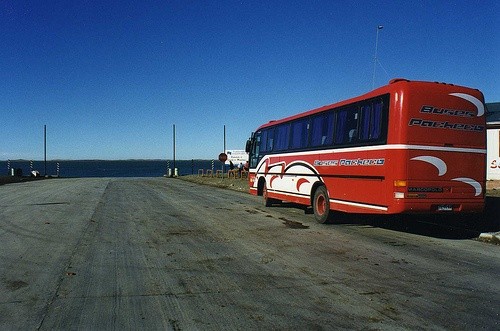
[245,78,487,225]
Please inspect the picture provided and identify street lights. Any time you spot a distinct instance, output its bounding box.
[373,25,384,88]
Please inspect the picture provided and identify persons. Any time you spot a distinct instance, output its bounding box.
[230,160,234,177]
[244,161,249,176]
[238,163,244,178]
